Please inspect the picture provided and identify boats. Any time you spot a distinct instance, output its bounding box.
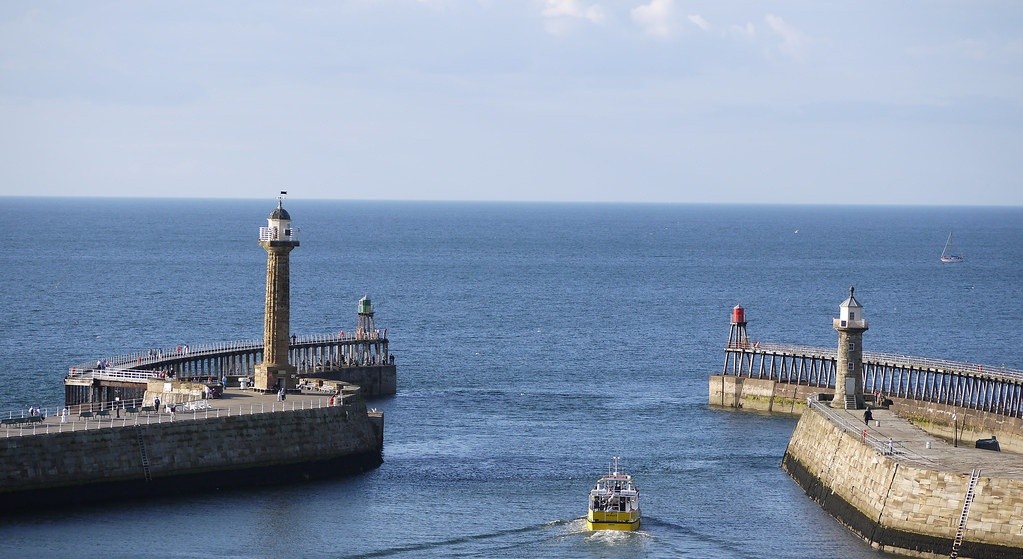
[586,456,642,532]
[940,230,965,263]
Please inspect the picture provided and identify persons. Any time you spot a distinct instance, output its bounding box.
[340,331,344,341]
[62,407,68,423]
[290,333,296,345]
[137,348,162,365]
[875,390,881,403]
[278,388,285,402]
[753,341,761,348]
[863,406,873,425]
[177,343,189,356]
[155,397,160,413]
[291,353,394,369]
[152,368,175,378]
[594,497,599,511]
[330,395,335,405]
[171,406,177,420]
[194,374,227,399]
[28,406,41,416]
[97,359,109,373]
[248,377,254,387]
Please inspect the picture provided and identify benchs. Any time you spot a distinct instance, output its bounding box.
[1,415,42,428]
[299,383,314,390]
[140,406,157,414]
[96,410,110,419]
[78,412,94,420]
[125,407,139,416]
[320,385,334,393]
